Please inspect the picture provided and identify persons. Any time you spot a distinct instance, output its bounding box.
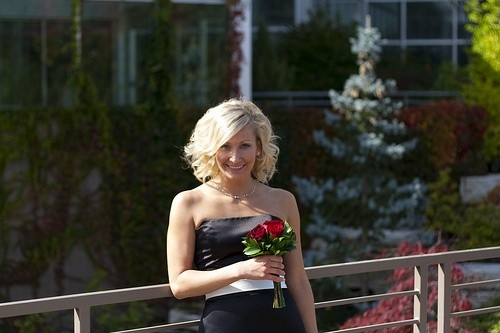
[167,98,318,333]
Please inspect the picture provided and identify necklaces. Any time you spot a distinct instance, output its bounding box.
[213,178,257,198]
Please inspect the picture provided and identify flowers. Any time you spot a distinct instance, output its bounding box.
[241,219,296,308]
